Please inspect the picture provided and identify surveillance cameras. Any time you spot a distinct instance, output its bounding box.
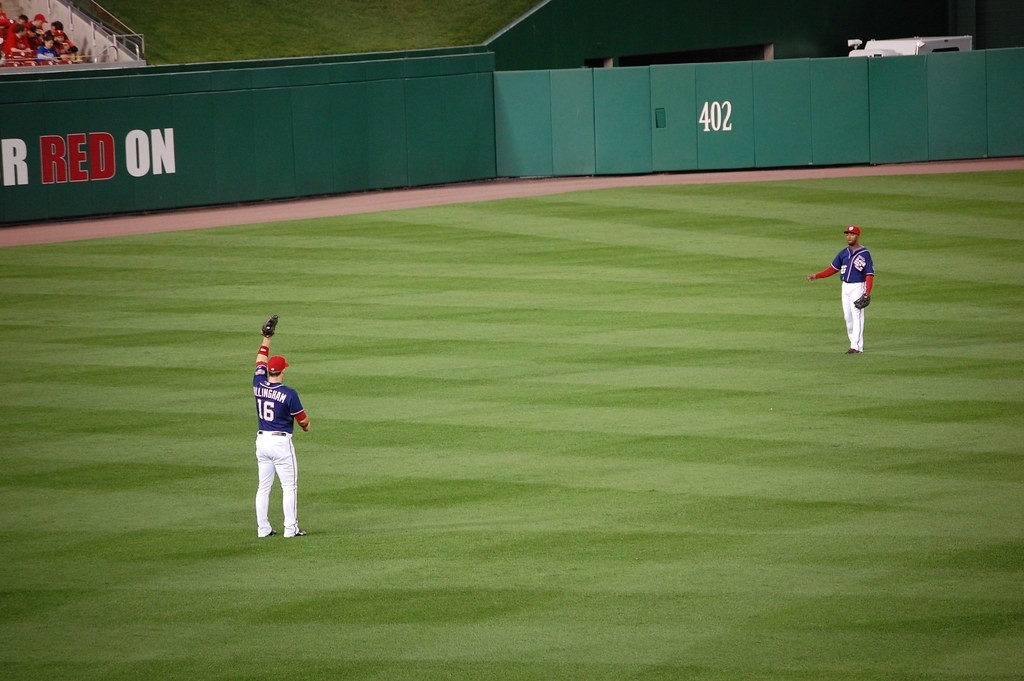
[848,40,862,47]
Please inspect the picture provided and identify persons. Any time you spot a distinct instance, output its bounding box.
[0,2,80,67]
[805,226,875,355]
[253,314,310,537]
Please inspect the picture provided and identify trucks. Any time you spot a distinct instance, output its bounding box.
[849,35,973,57]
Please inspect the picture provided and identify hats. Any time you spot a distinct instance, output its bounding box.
[268,355,289,373]
[54,30,65,36]
[844,225,861,236]
[35,13,48,23]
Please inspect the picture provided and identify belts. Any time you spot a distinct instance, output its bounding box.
[259,431,286,436]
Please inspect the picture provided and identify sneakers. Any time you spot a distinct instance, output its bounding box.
[296,530,306,536]
[845,348,864,354]
[267,530,276,537]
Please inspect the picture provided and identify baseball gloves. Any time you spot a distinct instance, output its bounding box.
[853,292,872,310]
[261,314,280,339]
